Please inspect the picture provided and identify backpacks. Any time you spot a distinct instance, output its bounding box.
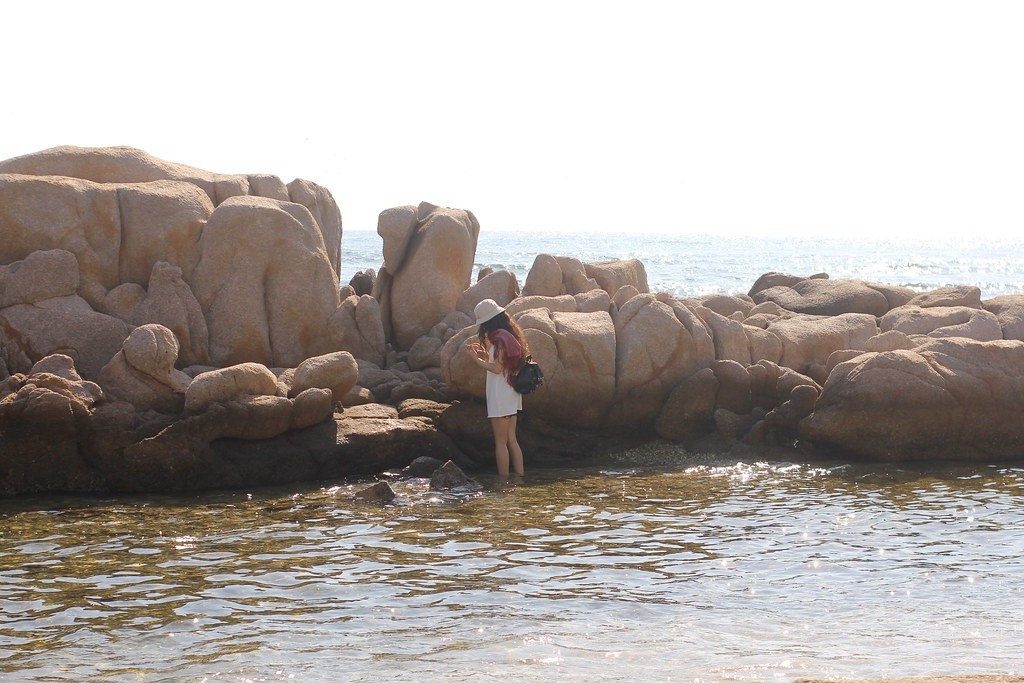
[506,354,544,394]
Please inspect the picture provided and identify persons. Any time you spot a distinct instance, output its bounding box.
[463,299,530,485]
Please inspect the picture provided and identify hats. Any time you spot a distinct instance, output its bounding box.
[474,298,506,325]
